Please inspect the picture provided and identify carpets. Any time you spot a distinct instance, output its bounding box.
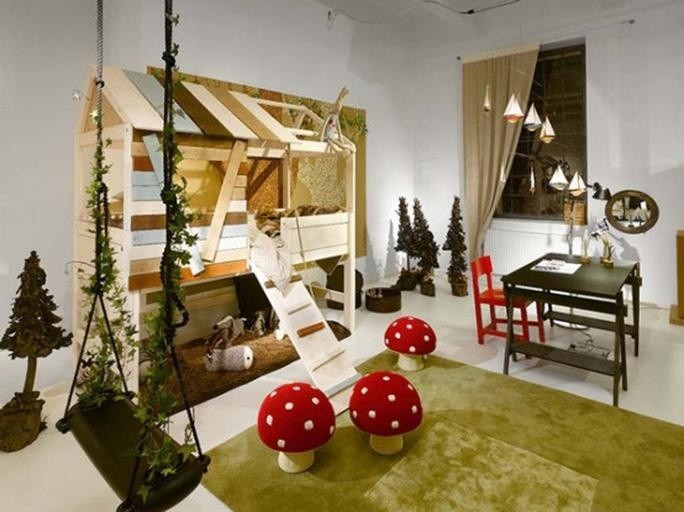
[137,321,352,423]
[199,349,684,512]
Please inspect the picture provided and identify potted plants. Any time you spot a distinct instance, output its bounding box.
[392,196,419,291]
[0,251,74,453]
[442,195,470,297]
[409,197,440,297]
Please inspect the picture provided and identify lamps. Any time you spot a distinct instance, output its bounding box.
[483,84,611,260]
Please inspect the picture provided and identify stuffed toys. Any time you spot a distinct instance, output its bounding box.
[319,86,358,159]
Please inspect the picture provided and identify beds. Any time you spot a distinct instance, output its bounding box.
[72,208,349,296]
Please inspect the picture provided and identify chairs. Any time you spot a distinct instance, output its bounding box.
[470,256,545,359]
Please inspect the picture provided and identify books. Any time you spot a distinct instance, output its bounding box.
[547,257,567,268]
[533,258,561,270]
[530,260,582,276]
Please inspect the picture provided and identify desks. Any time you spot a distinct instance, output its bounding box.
[501,253,642,407]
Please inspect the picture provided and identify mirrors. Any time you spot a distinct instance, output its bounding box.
[605,190,658,233]
